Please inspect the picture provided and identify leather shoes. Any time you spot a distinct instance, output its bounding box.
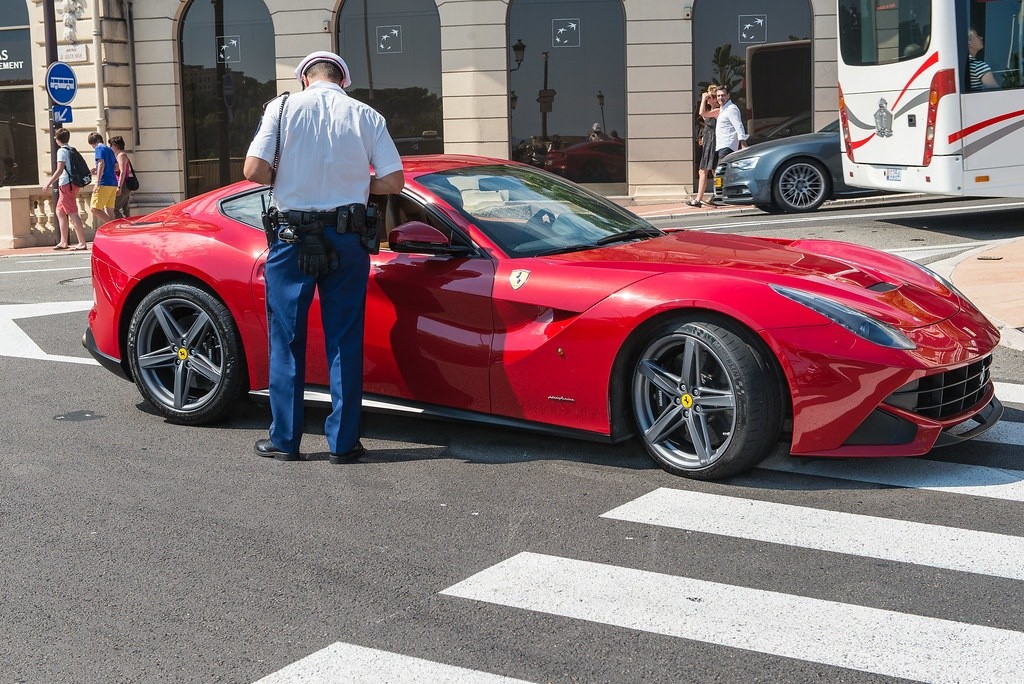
[254,438,300,461]
[329,442,365,464]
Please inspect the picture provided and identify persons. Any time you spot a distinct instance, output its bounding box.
[87,131,119,222]
[518,133,561,168]
[243,50,404,464]
[42,128,88,251]
[108,136,132,219]
[586,122,625,143]
[422,181,462,245]
[968,27,1001,90]
[685,85,750,208]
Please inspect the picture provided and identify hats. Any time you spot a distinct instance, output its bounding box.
[294,51,352,89]
[593,123,601,131]
[530,136,537,140]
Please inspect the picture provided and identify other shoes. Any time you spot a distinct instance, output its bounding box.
[686,200,701,207]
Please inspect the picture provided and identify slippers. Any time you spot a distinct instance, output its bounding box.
[71,247,87,251]
[54,245,70,250]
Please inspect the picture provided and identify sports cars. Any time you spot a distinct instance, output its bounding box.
[83,154,1003,481]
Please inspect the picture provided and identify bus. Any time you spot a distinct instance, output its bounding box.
[835,0,1023,199]
[745,39,813,138]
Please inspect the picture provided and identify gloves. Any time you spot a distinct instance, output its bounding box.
[298,220,338,279]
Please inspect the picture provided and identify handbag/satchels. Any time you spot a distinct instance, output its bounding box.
[127,177,139,191]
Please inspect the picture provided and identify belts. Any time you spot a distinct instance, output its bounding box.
[318,213,337,227]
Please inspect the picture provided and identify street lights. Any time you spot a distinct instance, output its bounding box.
[596,90,606,133]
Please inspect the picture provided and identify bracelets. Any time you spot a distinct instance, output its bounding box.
[118,187,120,188]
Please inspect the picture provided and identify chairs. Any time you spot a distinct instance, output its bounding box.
[385,193,426,244]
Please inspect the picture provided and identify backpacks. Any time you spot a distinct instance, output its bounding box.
[61,146,92,187]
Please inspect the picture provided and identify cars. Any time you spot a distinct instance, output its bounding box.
[392,130,443,155]
[713,117,913,214]
[545,140,627,183]
[749,110,811,145]
